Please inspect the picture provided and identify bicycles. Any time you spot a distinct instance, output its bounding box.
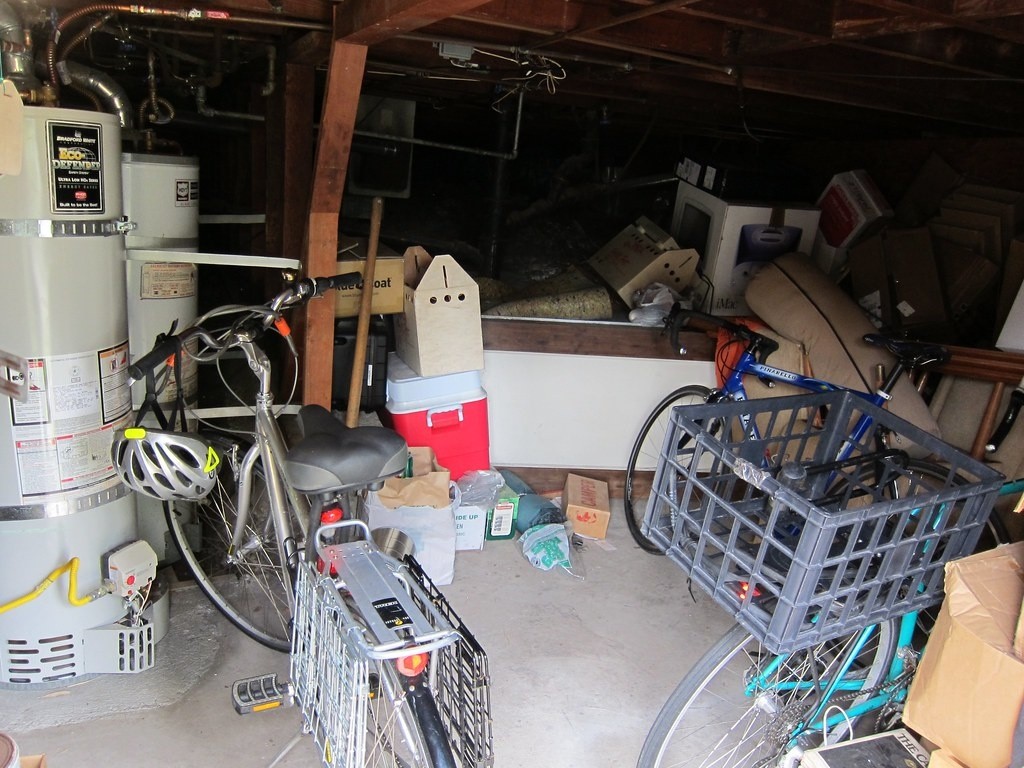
[622,303,1006,648]
[631,386,1024,768]
[129,269,498,768]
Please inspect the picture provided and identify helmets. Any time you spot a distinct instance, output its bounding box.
[110,428,220,501]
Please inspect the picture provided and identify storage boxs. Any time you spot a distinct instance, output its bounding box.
[587,216,681,290]
[809,150,1024,352]
[453,506,485,550]
[640,391,1007,655]
[385,351,489,481]
[392,245,484,379]
[334,237,404,318]
[670,180,822,317]
[901,541,1024,768]
[331,314,389,412]
[484,483,520,541]
[562,473,611,540]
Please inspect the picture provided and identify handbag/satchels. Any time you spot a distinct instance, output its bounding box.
[367,481,462,587]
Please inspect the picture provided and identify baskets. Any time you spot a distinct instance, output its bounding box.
[640,391,1007,655]
